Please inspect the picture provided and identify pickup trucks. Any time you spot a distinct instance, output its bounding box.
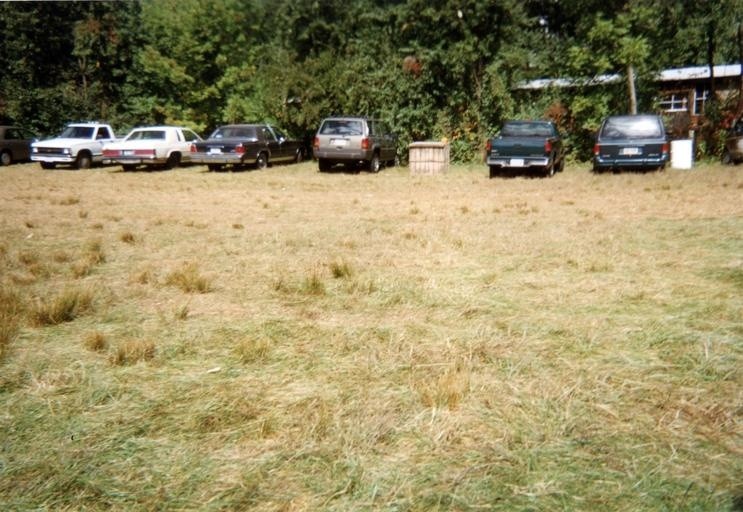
[484,119,569,175]
[28,123,127,169]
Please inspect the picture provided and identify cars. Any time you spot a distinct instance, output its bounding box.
[720,113,743,165]
[0,125,41,166]
[98,125,210,171]
[188,121,311,168]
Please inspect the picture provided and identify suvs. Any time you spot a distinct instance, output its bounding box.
[311,116,399,171]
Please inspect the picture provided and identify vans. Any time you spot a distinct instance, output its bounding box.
[592,114,669,174]
